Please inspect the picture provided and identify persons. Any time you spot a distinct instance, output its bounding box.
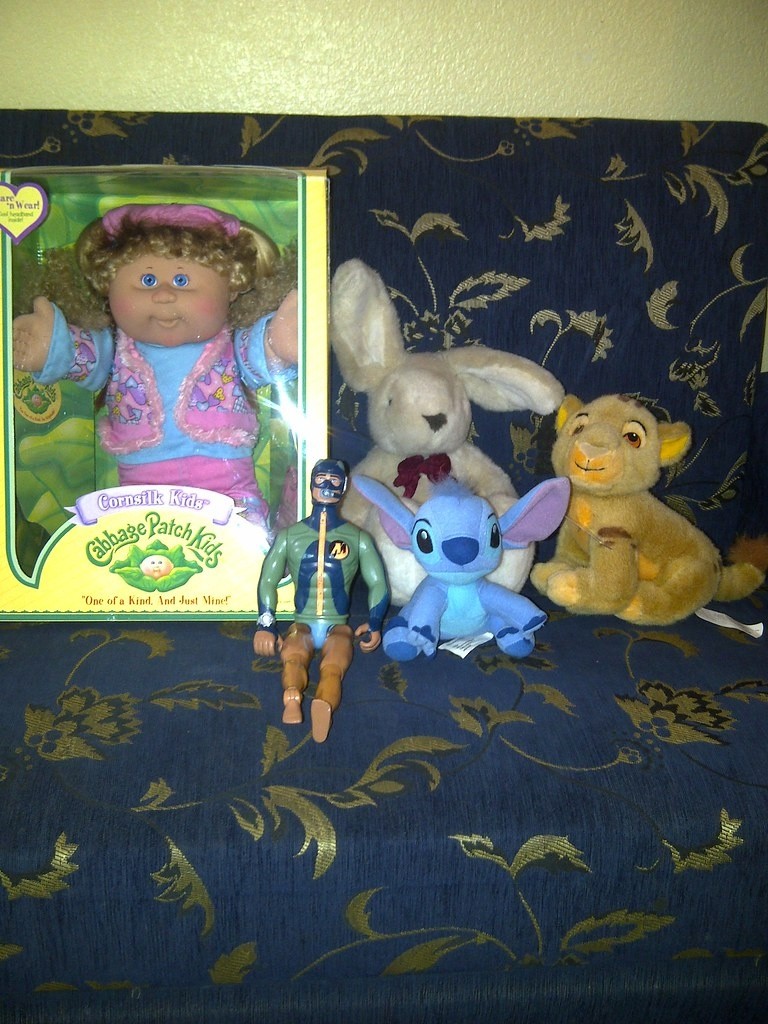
[12,203,300,531]
[254,459,390,742]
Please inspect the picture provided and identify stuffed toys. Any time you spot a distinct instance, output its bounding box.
[351,474,573,659]
[529,394,765,626]
[328,257,565,593]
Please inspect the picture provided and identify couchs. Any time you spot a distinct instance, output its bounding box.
[0,108,768,1024]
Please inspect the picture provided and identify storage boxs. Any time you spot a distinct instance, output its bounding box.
[1,166,340,628]
